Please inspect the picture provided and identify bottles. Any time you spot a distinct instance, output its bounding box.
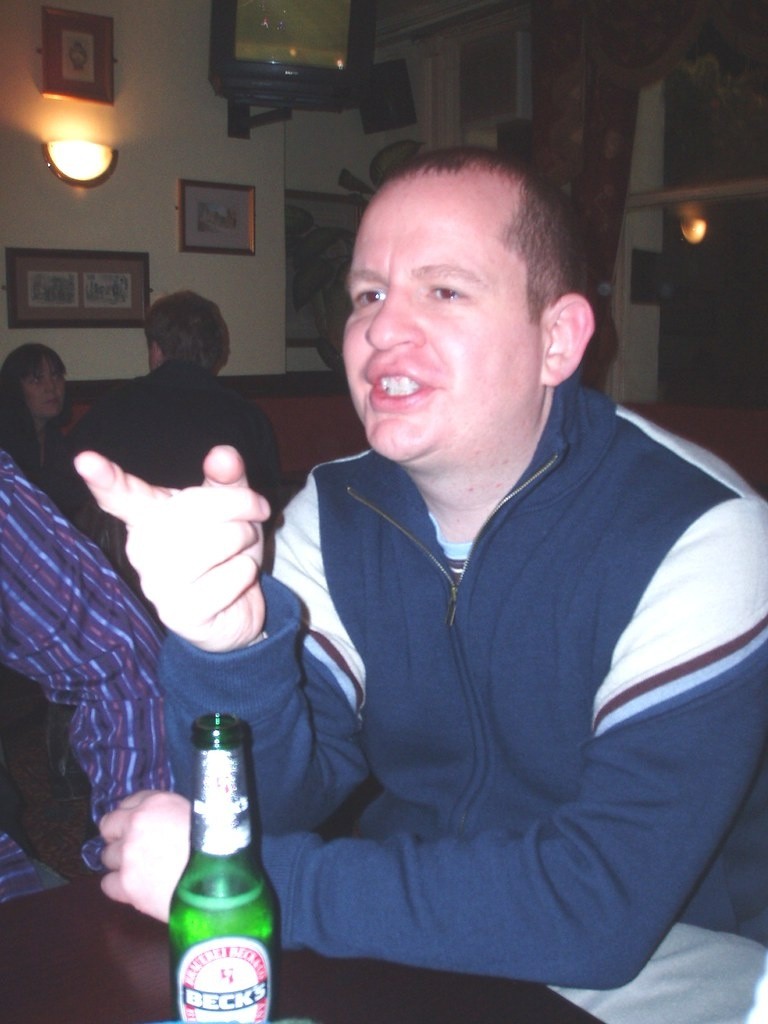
[168,714,281,1024]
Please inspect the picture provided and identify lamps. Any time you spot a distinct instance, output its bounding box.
[42,139,119,188]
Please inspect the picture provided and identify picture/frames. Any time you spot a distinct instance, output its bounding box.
[40,6,115,107]
[177,179,256,255]
[5,246,151,328]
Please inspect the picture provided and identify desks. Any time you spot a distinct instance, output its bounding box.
[0,877,608,1024]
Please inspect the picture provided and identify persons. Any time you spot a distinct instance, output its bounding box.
[0,449,173,907]
[0,344,89,529]
[62,291,281,580]
[73,148,768,1024]
